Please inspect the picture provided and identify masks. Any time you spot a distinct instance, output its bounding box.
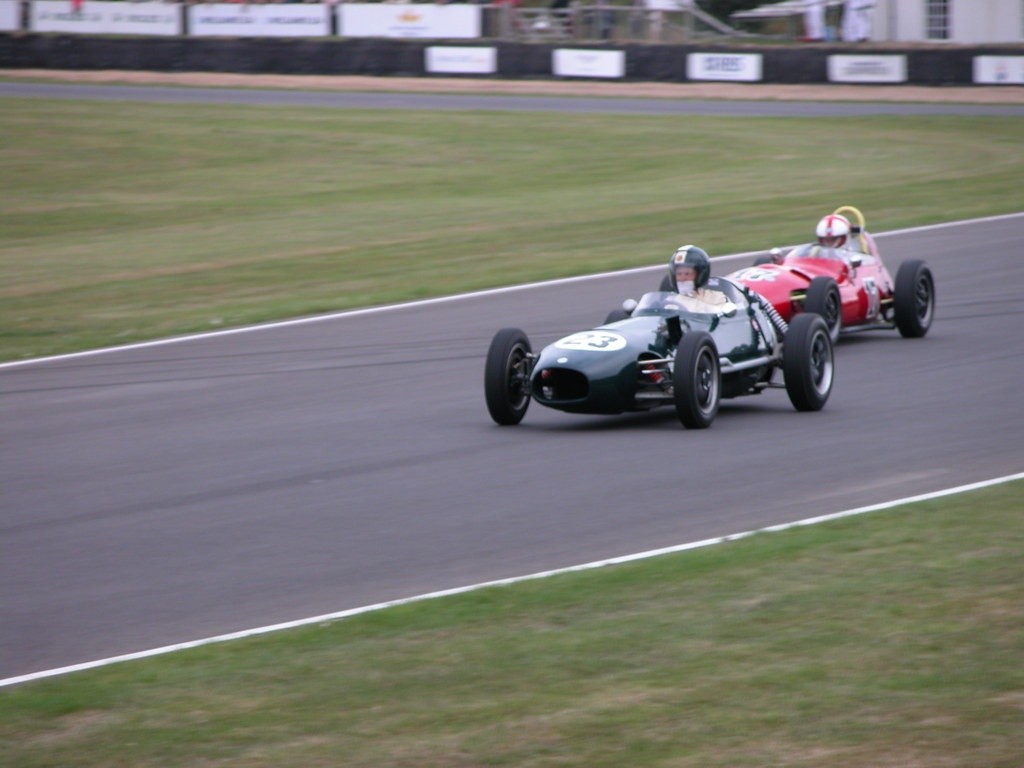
[676,281,696,293]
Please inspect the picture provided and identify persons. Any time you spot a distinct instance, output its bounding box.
[816,214,852,250]
[664,245,732,314]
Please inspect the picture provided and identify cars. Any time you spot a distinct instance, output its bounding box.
[717,206,937,346]
[481,243,836,429]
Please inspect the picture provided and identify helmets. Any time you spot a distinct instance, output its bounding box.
[815,213,851,249]
[667,245,711,292]
